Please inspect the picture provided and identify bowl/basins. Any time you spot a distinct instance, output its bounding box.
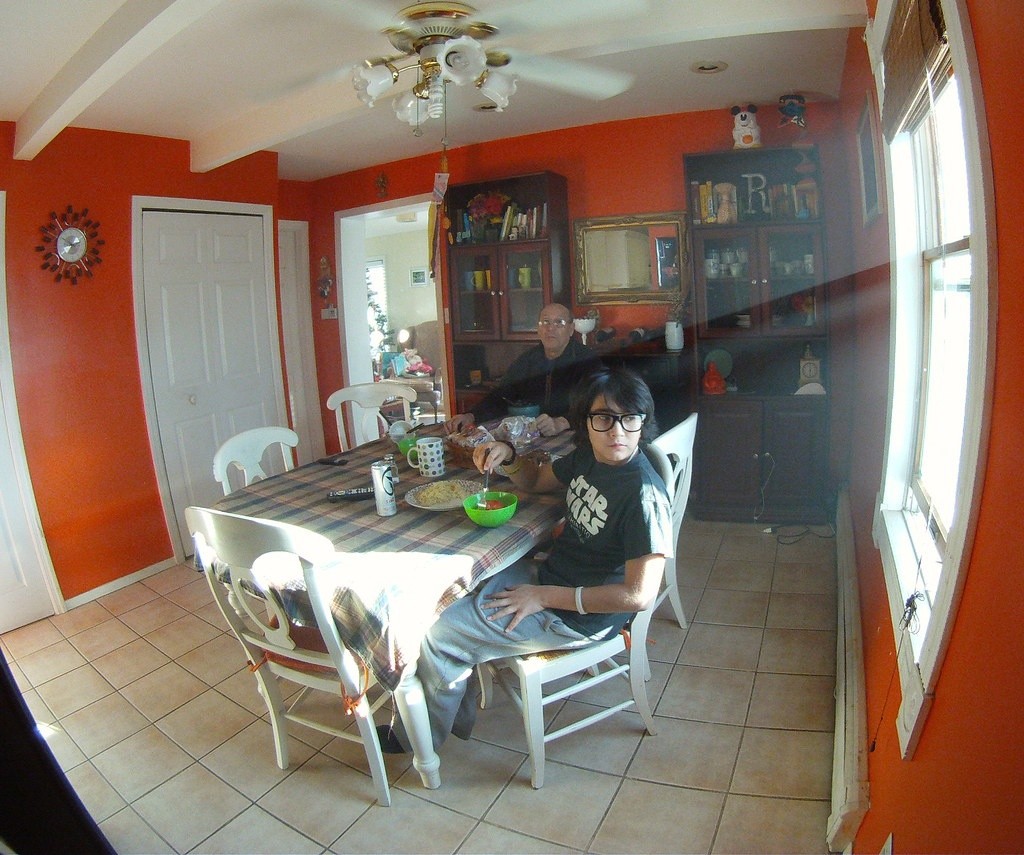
[448,440,490,468]
[463,492,518,528]
[507,404,540,419]
[388,421,422,458]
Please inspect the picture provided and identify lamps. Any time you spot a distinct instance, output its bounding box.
[350,1,520,173]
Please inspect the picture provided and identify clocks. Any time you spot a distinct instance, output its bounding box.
[55,227,88,263]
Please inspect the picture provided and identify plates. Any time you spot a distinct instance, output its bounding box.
[734,313,784,329]
[494,448,562,477]
[405,480,486,511]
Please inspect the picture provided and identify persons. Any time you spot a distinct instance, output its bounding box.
[443,303,603,436]
[376,368,674,754]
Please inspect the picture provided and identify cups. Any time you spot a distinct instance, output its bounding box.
[705,242,815,280]
[469,369,482,387]
[407,437,446,477]
[458,266,532,291]
[665,321,684,350]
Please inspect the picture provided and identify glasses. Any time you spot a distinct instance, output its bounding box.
[587,414,647,432]
[537,319,571,328]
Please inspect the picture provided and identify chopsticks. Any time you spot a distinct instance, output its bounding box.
[407,422,424,433]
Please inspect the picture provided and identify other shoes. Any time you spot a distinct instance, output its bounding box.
[376,725,406,754]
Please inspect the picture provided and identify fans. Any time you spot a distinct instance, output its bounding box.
[315,1,634,103]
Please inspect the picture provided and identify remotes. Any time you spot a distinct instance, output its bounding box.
[327,487,374,503]
[317,458,348,465]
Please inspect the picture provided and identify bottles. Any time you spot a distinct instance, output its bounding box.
[385,453,400,484]
[596,327,664,347]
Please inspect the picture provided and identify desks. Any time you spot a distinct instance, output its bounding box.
[193,424,579,789]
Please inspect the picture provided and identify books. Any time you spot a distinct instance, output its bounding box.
[690,176,818,224]
[500,202,547,242]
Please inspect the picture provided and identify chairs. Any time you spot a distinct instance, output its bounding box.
[184,505,405,807]
[212,425,300,497]
[326,384,416,452]
[475,550,657,789]
[636,411,698,678]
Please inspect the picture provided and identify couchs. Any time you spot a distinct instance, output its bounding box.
[380,320,443,424]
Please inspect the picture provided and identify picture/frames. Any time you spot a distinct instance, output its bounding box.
[409,265,430,288]
[854,88,884,230]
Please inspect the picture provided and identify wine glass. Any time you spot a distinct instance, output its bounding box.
[573,319,595,347]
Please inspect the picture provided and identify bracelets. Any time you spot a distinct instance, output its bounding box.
[496,440,521,475]
[575,587,587,614]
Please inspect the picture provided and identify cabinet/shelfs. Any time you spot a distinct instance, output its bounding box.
[443,172,579,418]
[681,147,835,526]
